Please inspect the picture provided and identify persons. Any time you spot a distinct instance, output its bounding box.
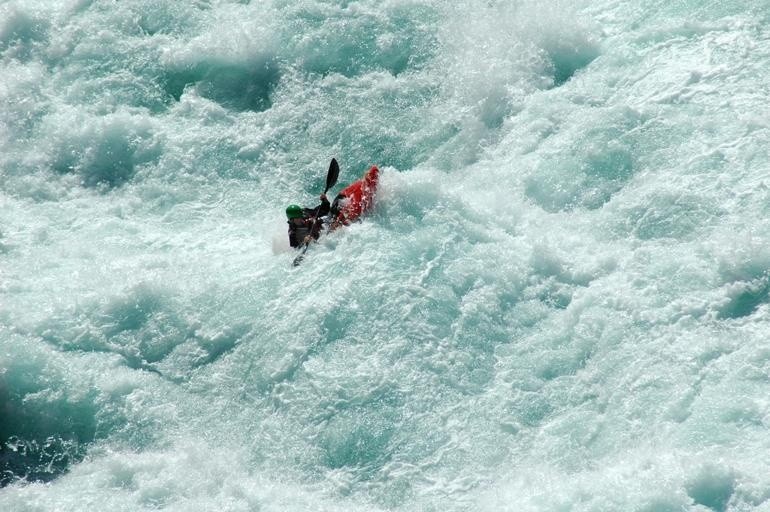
[285,193,331,249]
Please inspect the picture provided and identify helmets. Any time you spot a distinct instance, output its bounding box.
[286,204,304,219]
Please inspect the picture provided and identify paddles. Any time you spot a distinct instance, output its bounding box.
[292,157,340,266]
